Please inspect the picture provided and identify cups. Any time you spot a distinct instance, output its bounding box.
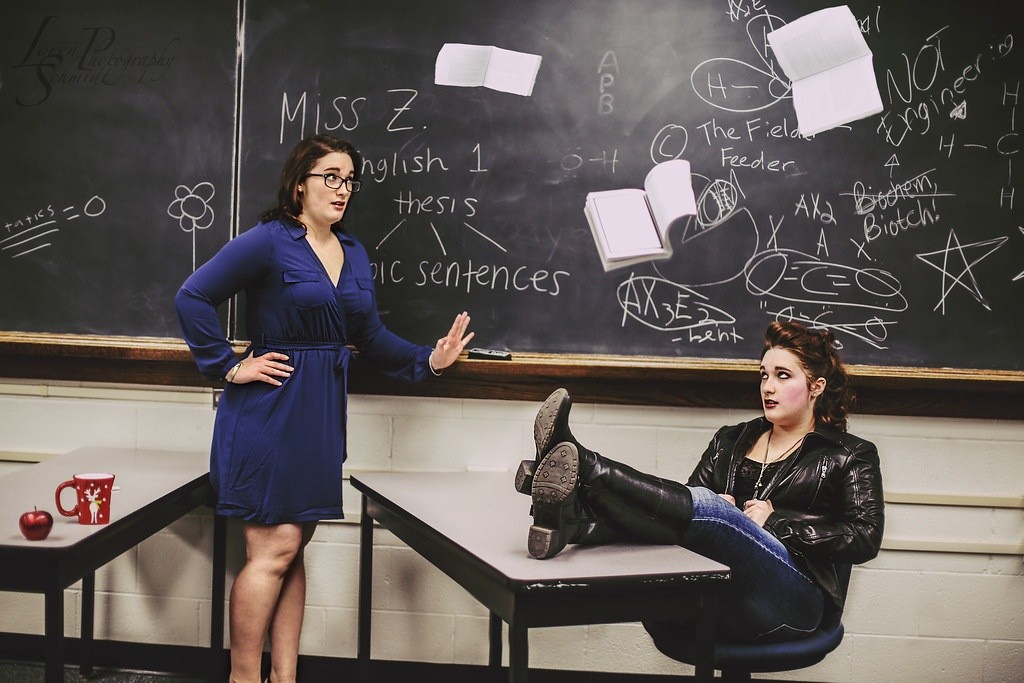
[55,473,115,524]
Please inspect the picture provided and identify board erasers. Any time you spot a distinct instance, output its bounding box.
[467,347,512,362]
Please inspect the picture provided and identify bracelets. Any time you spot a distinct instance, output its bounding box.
[227,363,241,382]
[429,351,444,376]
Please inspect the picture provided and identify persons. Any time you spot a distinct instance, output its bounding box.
[175,136,474,683]
[515,320,884,643]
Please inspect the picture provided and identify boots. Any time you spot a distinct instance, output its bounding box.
[526,442,645,559]
[514,393,692,544]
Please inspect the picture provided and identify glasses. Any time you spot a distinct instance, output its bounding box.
[306,173,363,193]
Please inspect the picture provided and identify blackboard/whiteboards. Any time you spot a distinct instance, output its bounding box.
[1,2,1022,422]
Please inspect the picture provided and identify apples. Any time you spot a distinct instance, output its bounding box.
[20,506,53,540]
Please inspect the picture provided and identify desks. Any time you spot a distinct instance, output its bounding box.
[0,447,226,683]
[347,470,729,683]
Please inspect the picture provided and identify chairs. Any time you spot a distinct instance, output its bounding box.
[642,558,856,683]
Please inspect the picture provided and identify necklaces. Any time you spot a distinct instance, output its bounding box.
[753,419,814,499]
